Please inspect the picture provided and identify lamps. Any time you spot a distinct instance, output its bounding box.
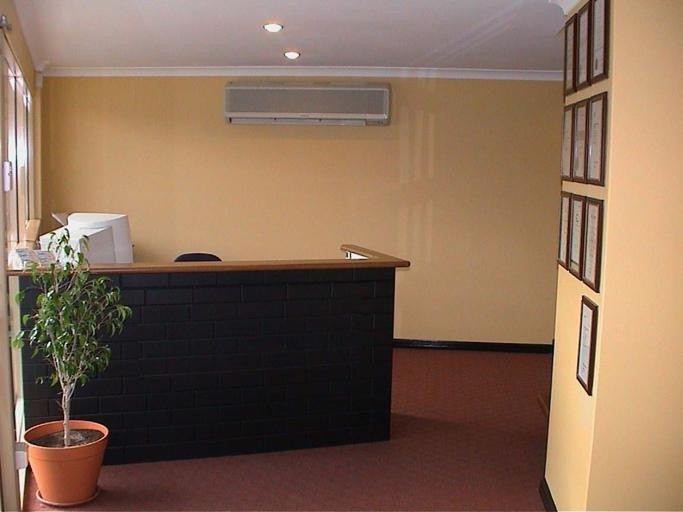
[174,253,220,261]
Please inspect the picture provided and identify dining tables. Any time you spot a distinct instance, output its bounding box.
[12,228,132,506]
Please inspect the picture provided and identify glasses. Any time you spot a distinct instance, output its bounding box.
[262,23,283,32]
[284,51,300,60]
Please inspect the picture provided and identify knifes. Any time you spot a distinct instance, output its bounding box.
[226,81,393,127]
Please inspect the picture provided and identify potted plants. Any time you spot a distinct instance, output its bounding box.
[565,13,575,95]
[590,0,610,85]
[585,90,606,186]
[575,1,590,91]
[582,196,604,292]
[561,104,572,182]
[557,190,570,270]
[567,194,585,280]
[576,296,598,395]
[571,98,588,185]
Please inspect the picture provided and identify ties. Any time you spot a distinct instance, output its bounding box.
[40,211,134,265]
